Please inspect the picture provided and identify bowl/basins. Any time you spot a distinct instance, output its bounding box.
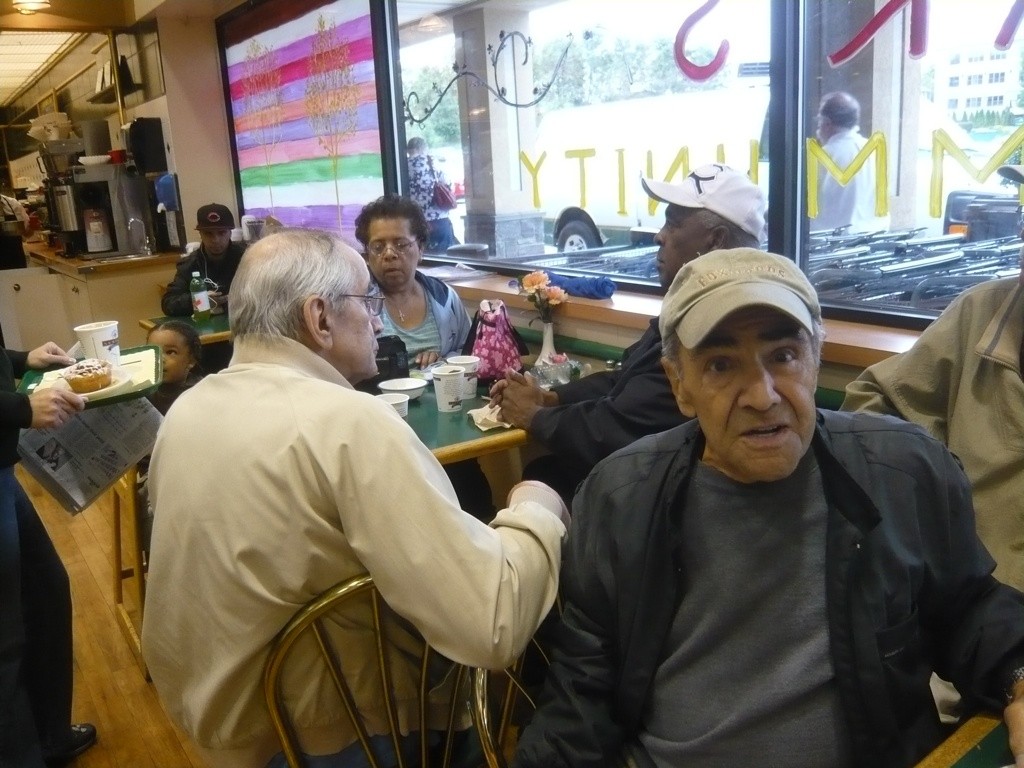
[378,378,429,400]
[41,120,72,142]
[375,393,410,418]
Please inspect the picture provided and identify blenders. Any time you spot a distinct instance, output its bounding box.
[65,164,140,259]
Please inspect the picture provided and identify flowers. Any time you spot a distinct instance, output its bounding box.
[517,271,568,325]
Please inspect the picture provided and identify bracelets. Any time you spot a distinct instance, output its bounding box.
[1004,667,1024,705]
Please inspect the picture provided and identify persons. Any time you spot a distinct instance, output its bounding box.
[139,224,572,768]
[355,193,494,526]
[2,330,97,767]
[810,90,890,233]
[508,243,1024,768]
[406,136,459,254]
[835,258,1023,768]
[0,194,37,269]
[139,321,204,554]
[482,162,770,476]
[162,201,254,374]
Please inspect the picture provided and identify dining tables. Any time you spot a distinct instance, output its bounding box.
[398,363,528,466]
[138,316,233,345]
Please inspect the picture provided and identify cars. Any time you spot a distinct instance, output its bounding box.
[537,97,765,254]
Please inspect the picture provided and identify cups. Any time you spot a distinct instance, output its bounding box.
[552,363,570,386]
[432,367,465,412]
[446,356,480,398]
[530,364,557,396]
[73,319,120,366]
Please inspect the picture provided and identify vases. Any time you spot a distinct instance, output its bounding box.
[534,323,559,366]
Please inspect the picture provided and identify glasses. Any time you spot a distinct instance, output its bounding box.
[323,290,385,316]
[365,236,420,256]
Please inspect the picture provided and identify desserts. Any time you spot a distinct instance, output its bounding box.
[62,356,114,393]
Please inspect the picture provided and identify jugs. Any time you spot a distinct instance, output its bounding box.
[76,199,112,253]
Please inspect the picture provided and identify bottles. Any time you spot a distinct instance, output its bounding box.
[617,362,622,369]
[606,360,615,371]
[90,212,106,250]
[190,271,211,323]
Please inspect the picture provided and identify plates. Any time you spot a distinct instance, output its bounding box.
[51,369,130,398]
[78,155,111,164]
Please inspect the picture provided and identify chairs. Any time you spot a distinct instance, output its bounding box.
[110,463,151,656]
[260,573,471,768]
[469,612,550,768]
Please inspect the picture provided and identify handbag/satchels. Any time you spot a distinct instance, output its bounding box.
[460,298,529,378]
[435,181,457,210]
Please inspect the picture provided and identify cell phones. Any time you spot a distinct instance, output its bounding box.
[211,284,229,295]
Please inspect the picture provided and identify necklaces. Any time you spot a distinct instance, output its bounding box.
[384,287,407,323]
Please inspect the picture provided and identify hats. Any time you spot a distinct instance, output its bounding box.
[661,245,822,349]
[194,203,235,230]
[642,161,768,238]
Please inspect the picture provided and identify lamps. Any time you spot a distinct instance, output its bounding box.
[12,0,52,15]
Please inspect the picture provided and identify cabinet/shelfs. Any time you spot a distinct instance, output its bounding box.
[12,244,184,352]
